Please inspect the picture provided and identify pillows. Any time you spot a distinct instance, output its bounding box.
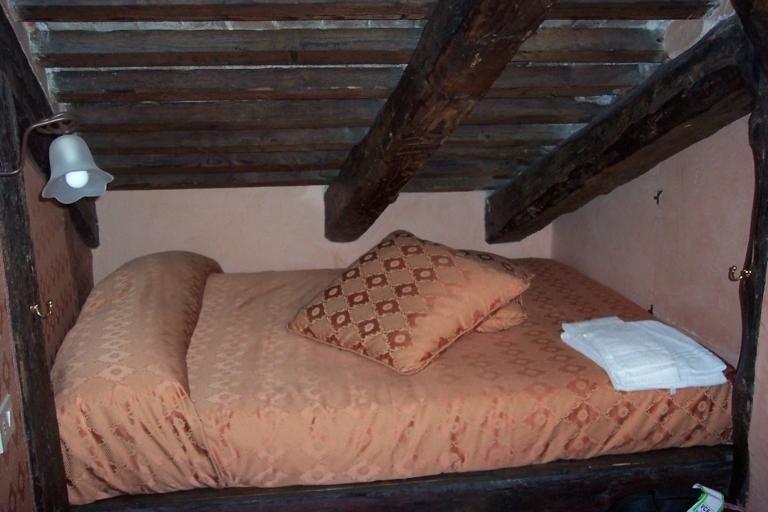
[285,228,537,377]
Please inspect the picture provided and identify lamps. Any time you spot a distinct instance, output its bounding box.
[1,110,114,206]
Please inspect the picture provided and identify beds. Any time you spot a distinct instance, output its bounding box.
[17,255,751,512]
